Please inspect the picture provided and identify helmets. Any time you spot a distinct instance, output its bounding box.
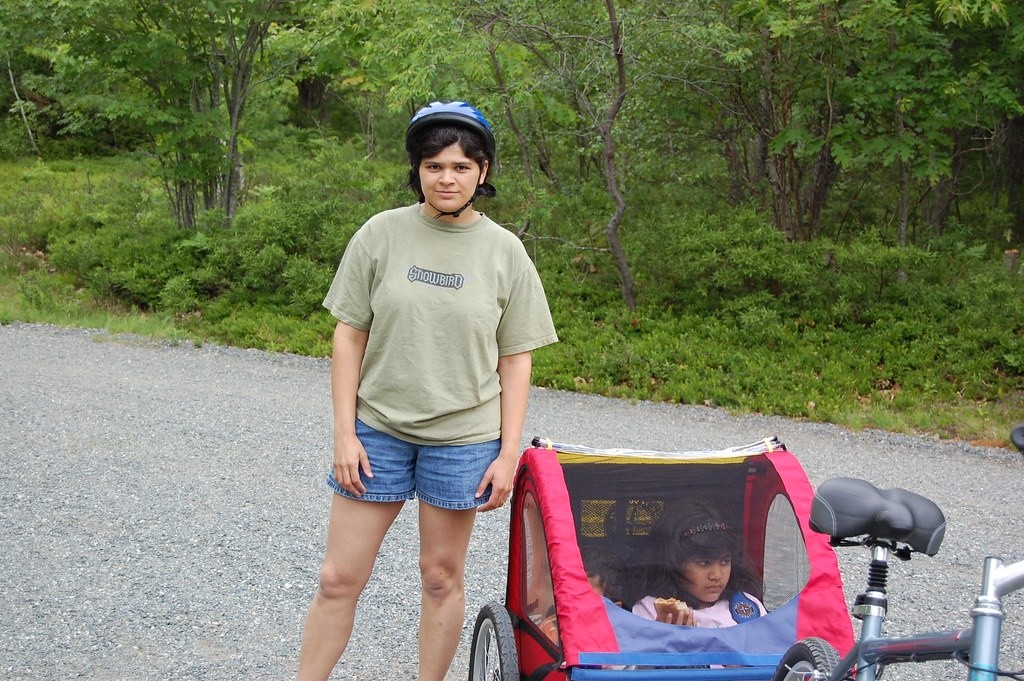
[406,99,496,165]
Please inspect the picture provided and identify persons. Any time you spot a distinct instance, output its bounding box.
[539,501,769,671]
[294,101,558,680]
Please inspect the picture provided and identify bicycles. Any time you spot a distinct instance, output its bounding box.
[775,425,1024,680]
